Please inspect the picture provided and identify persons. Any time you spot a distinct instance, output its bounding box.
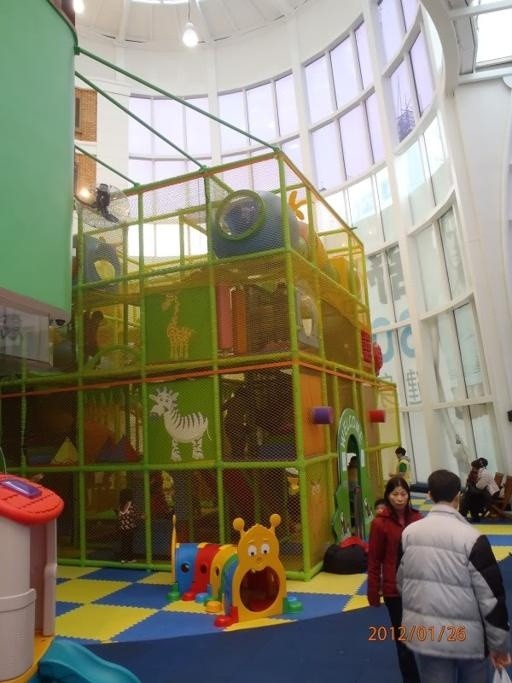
[472,460,499,519]
[459,458,488,522]
[367,477,424,682]
[273,282,287,295]
[29,439,73,503]
[373,500,386,597]
[222,387,249,462]
[84,310,104,369]
[398,469,512,682]
[390,447,412,510]
[92,184,120,223]
[117,489,144,564]
